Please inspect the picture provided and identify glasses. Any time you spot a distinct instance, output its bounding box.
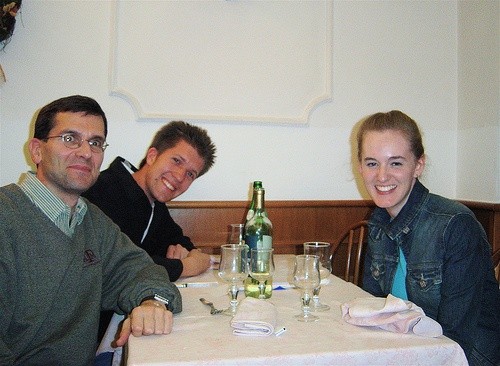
[41,133,109,154]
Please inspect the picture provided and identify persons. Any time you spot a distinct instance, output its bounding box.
[354,111,500,366]
[0,96,184,366]
[83,122,218,344]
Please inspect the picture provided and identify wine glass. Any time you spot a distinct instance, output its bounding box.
[304,242,332,312]
[218,244,249,315]
[293,255,321,322]
[250,247,275,302]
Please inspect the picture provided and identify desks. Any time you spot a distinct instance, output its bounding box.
[114,255,470,366]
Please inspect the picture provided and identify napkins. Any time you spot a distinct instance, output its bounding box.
[230,295,278,337]
[339,296,444,338]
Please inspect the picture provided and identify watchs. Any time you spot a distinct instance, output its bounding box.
[141,294,169,310]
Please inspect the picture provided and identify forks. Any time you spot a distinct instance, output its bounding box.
[200,298,226,315]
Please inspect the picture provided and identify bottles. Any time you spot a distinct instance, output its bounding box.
[243,181,273,284]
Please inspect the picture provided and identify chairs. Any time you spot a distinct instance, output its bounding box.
[330,219,370,286]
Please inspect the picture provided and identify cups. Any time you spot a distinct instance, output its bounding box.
[230,223,243,244]
[242,256,272,300]
